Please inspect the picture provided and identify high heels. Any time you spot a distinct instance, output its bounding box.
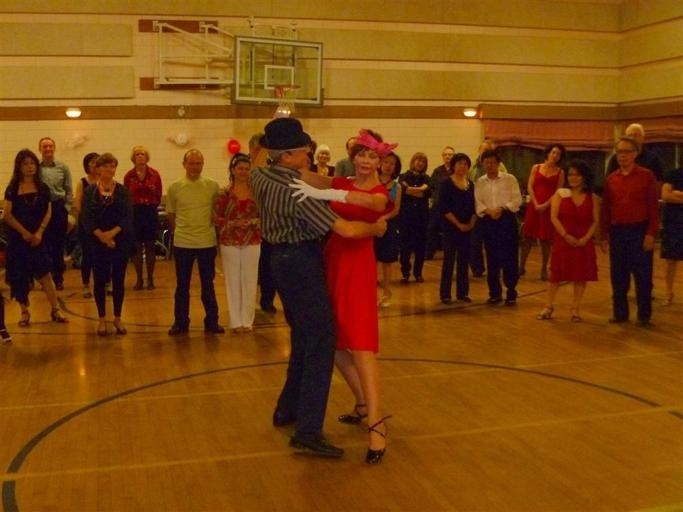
[113,319,127,334]
[18,310,30,326]
[338,404,367,423]
[51,308,69,323]
[365,415,392,464]
[98,320,108,336]
[379,289,393,307]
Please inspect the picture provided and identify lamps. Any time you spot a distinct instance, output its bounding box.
[66,107,83,119]
[463,107,477,118]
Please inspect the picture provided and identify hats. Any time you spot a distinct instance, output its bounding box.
[258,118,311,150]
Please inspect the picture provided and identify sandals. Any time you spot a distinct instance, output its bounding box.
[536,306,554,320]
[568,304,582,322]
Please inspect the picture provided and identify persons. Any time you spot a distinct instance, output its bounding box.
[605,123,661,182]
[40,138,73,290]
[247,133,278,314]
[166,149,224,336]
[662,151,683,307]
[246,118,388,461]
[0,292,11,343]
[518,144,565,280]
[375,151,402,308]
[335,138,359,178]
[212,153,262,334]
[310,145,335,177]
[600,138,661,327]
[429,146,455,259]
[475,151,523,306]
[537,160,601,322]
[80,153,132,336]
[398,152,432,284]
[289,127,399,465]
[75,153,117,298]
[5,149,71,326]
[468,140,508,277]
[439,152,474,304]
[124,145,162,291]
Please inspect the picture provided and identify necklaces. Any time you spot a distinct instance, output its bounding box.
[18,177,40,206]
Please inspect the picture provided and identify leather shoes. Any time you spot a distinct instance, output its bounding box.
[205,322,224,333]
[288,431,344,457]
[272,408,296,425]
[635,319,646,325]
[504,298,516,305]
[608,316,616,322]
[168,326,188,335]
[488,297,502,303]
